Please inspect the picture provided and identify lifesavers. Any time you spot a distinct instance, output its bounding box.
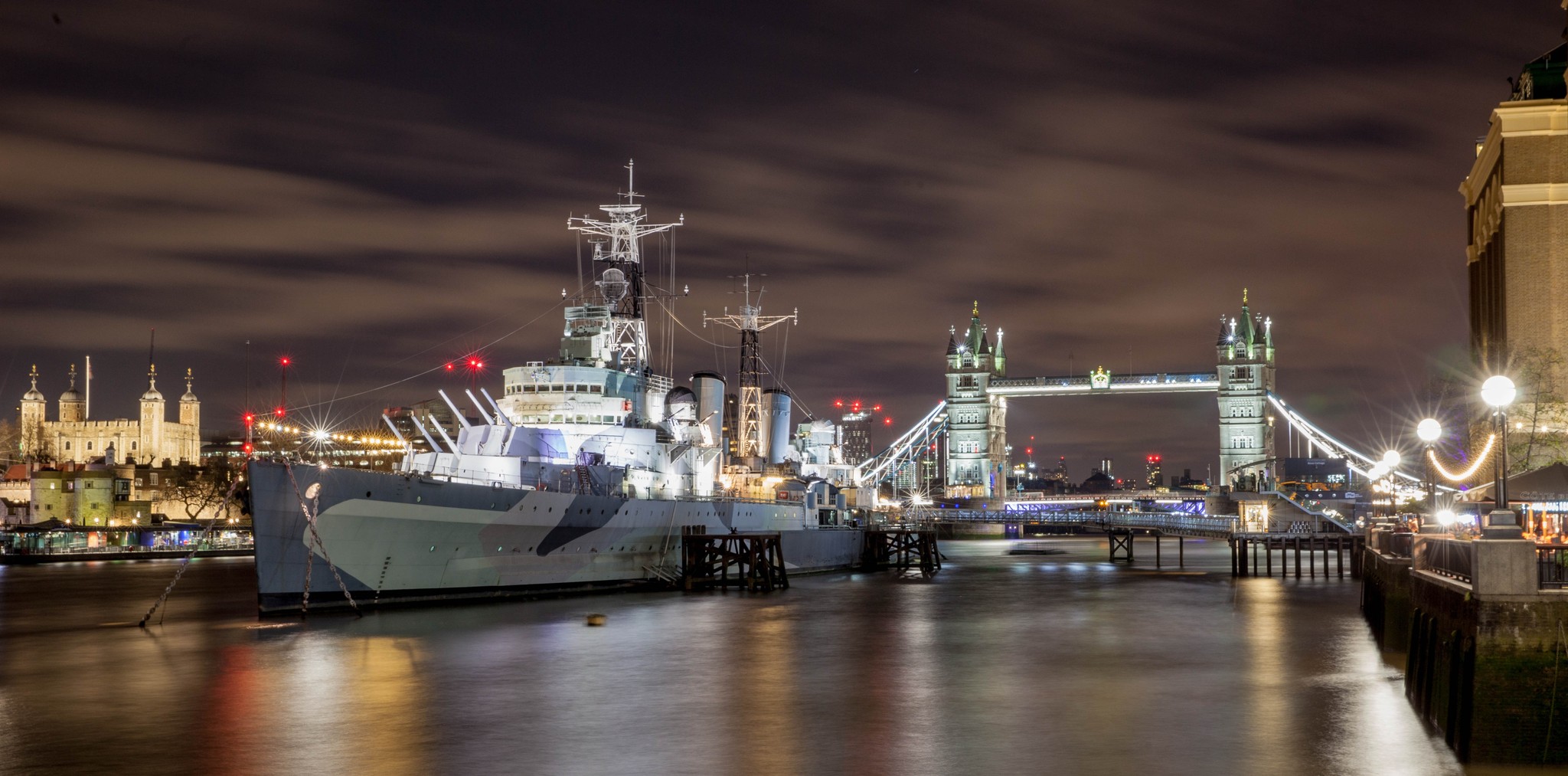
[792,496,798,501]
[537,484,546,492]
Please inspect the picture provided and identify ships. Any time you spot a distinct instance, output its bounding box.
[241,156,919,625]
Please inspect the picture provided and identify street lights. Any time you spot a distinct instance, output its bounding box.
[1415,417,1445,535]
[1367,468,1381,529]
[110,520,115,546]
[229,519,234,545]
[132,519,136,545]
[66,519,70,549]
[1374,461,1390,529]
[1383,450,1402,530]
[1480,374,1529,539]
[94,517,99,532]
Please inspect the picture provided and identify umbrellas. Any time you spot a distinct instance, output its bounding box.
[1453,461,1568,503]
